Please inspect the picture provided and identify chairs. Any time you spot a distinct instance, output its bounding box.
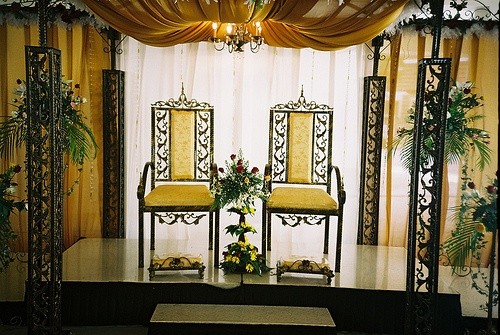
[136,82,220,269]
[261,85,347,272]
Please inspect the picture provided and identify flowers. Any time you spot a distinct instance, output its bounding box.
[208,148,275,278]
[1,74,99,196]
[442,170,499,310]
[0,163,28,271]
[386,78,495,171]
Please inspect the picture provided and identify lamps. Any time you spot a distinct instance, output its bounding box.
[209,22,264,53]
